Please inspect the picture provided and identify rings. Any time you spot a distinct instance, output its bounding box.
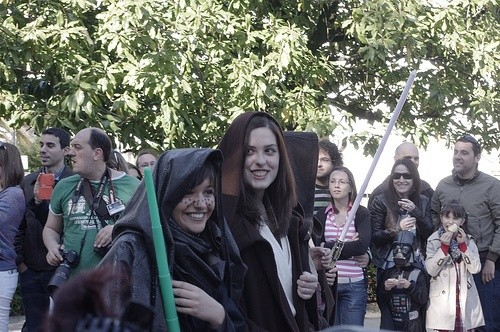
[405,205,408,207]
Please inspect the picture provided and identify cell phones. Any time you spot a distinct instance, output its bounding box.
[38,173,55,200]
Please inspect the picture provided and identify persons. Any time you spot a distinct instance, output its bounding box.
[0,111,500,332]
[213,113,319,332]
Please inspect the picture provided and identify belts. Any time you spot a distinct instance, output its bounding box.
[337,275,363,284]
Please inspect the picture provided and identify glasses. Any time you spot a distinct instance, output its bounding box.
[329,178,351,186]
[456,135,480,147]
[392,172,414,180]
[0,141,7,150]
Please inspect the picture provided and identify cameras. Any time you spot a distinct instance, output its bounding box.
[46,249,78,295]
